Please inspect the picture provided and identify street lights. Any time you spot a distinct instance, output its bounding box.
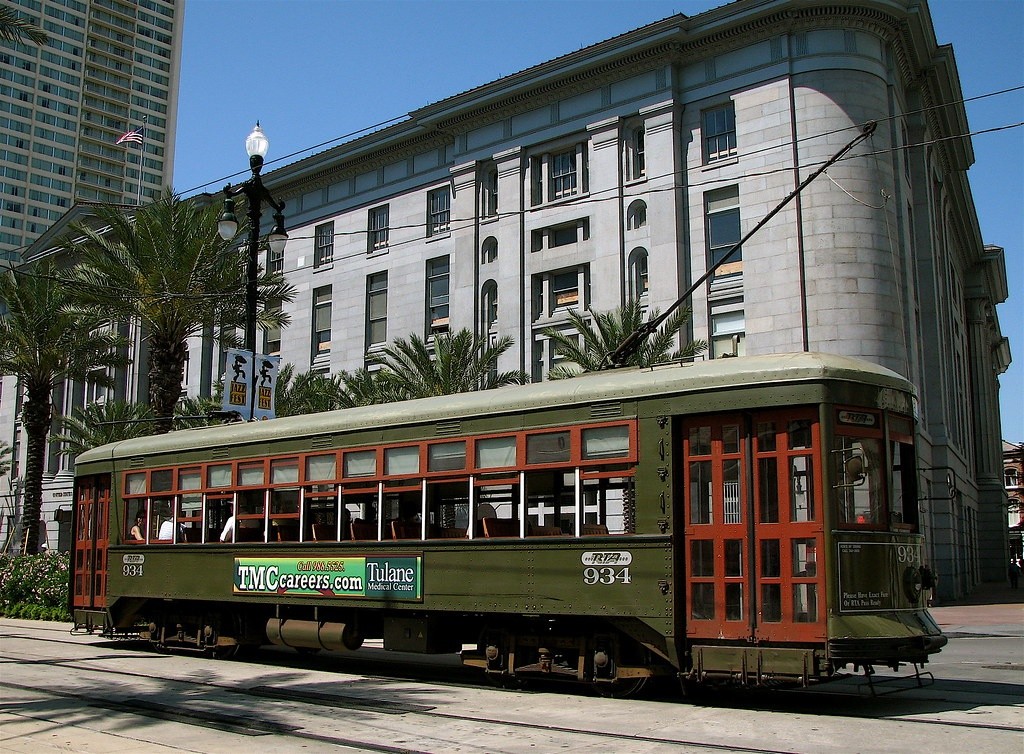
[217,121,289,420]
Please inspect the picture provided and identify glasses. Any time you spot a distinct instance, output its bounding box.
[138,515,145,519]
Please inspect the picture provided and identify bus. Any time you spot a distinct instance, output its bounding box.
[66,345,947,705]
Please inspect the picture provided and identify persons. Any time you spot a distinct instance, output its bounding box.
[130,508,144,540]
[220,495,260,542]
[158,517,186,541]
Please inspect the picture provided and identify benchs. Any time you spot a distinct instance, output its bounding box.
[126,516,608,544]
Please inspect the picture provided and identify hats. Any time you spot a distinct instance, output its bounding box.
[227,498,234,503]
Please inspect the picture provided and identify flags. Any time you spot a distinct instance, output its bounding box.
[116,126,143,144]
